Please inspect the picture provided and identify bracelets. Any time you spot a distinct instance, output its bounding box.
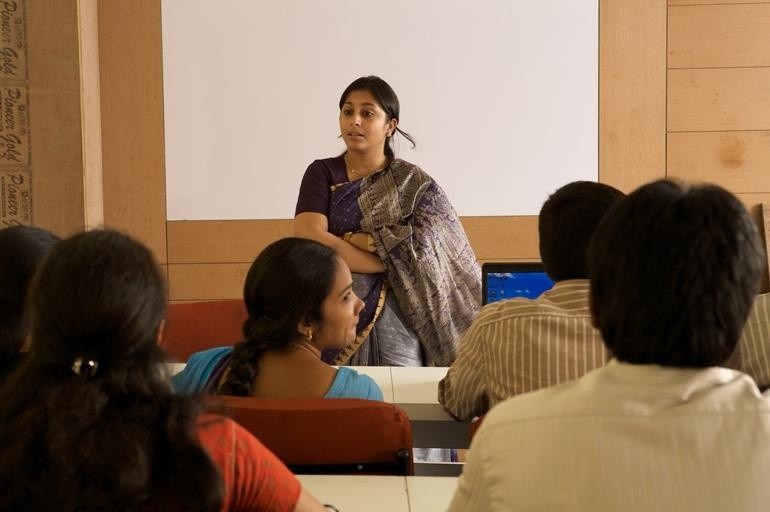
[341,230,353,243]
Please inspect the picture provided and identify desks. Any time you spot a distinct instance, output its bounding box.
[289,472,459,512]
[337,366,482,473]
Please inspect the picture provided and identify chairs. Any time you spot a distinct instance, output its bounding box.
[199,391,414,477]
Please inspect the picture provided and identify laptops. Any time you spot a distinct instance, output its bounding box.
[482,262,557,307]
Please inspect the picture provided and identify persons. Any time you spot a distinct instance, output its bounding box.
[437,180,625,422]
[0,222,65,387]
[0,224,333,510]
[172,238,385,407]
[291,73,486,465]
[446,178,770,510]
[730,201,770,395]
